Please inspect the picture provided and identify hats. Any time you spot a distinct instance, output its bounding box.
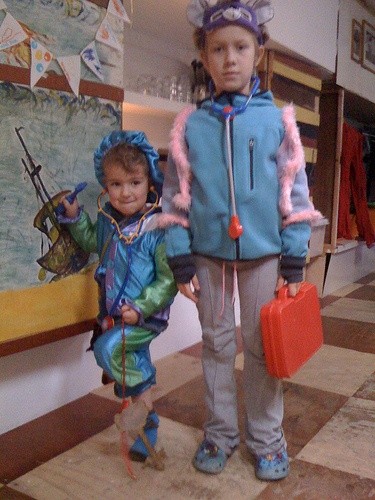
[185,0,274,45]
[93,130,164,205]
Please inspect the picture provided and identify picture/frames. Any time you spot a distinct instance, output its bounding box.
[361,19,375,73]
[350,19,362,65]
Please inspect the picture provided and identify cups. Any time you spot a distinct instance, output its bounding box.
[128,74,192,102]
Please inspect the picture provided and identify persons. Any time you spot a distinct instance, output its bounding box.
[56,130,180,462]
[163,0,324,480]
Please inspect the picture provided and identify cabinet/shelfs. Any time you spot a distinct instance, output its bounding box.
[123,47,375,254]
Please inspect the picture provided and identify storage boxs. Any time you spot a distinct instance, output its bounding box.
[260,282,324,380]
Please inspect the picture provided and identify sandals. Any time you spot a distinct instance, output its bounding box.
[254,448,289,482]
[193,441,229,475]
